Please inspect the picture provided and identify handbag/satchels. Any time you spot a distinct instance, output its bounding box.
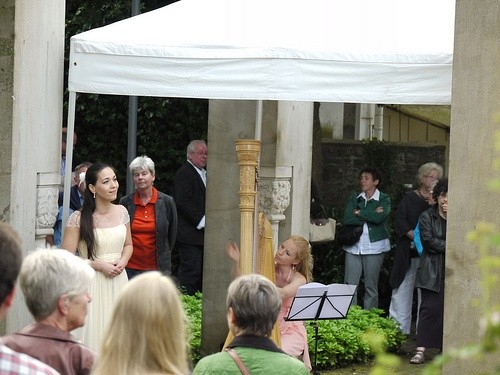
[309,202,336,244]
[413,209,432,257]
[336,225,363,245]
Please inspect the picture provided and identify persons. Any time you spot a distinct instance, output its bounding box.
[0,223,61,375]
[0,243,97,375]
[193,273,313,375]
[91,271,193,375]
[49,139,448,366]
[225,235,315,375]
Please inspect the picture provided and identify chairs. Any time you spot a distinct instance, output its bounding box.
[414,219,425,337]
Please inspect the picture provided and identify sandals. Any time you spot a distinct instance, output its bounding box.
[409,350,424,364]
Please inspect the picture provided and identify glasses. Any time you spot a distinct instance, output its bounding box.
[83,292,92,303]
[425,175,439,181]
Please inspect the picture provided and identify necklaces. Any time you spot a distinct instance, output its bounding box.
[277,268,293,284]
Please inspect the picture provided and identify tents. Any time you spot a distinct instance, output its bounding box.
[59,0,455,240]
